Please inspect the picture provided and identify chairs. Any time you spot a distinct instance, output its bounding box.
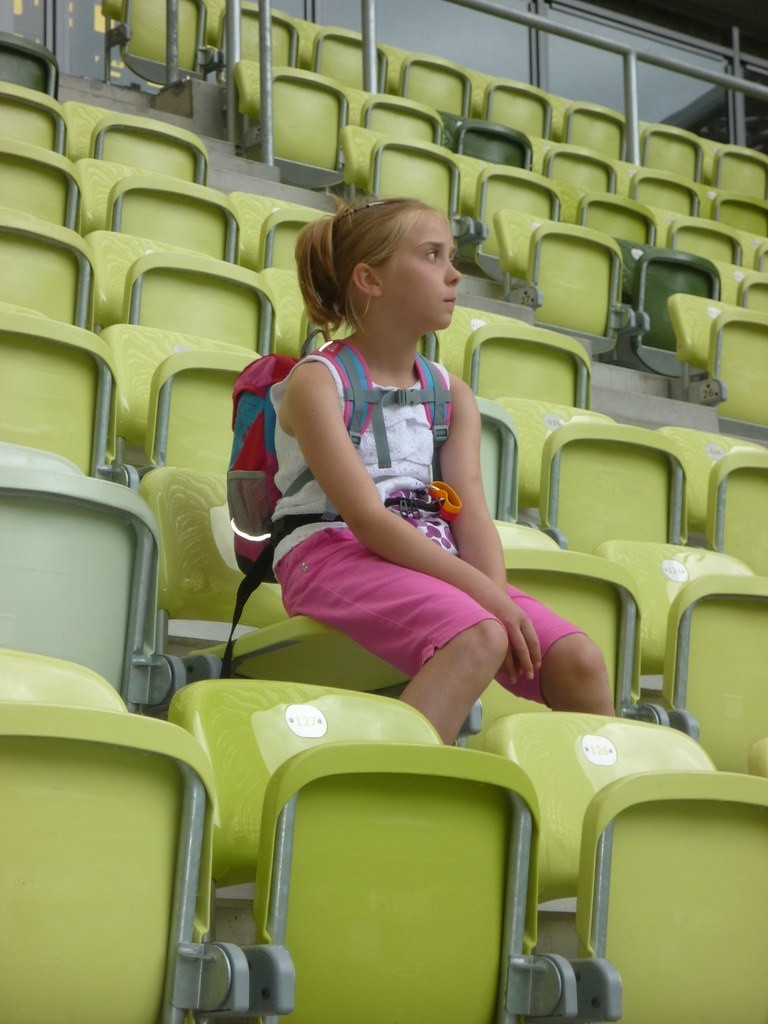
[0,0,768,1024]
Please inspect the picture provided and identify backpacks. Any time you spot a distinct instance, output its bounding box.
[227,339,453,582]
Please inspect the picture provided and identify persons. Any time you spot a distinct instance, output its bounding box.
[271,198,616,747]
